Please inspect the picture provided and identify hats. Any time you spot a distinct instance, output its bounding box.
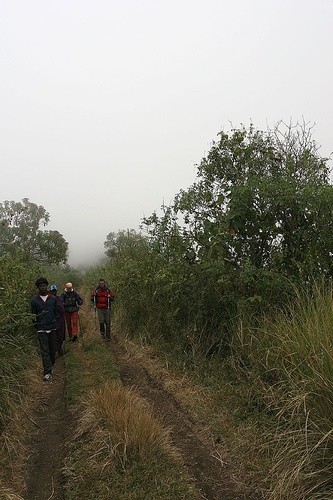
[35,277,48,287]
[66,282,73,288]
[50,284,57,290]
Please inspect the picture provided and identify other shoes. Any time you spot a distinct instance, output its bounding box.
[58,347,63,356]
[69,336,77,342]
[101,334,105,339]
[106,335,111,340]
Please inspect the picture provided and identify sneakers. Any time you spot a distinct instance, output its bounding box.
[43,373,52,381]
[51,367,56,374]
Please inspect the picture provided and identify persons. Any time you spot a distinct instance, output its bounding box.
[60,282,84,342]
[91,278,115,341]
[30,276,58,381]
[49,284,65,355]
